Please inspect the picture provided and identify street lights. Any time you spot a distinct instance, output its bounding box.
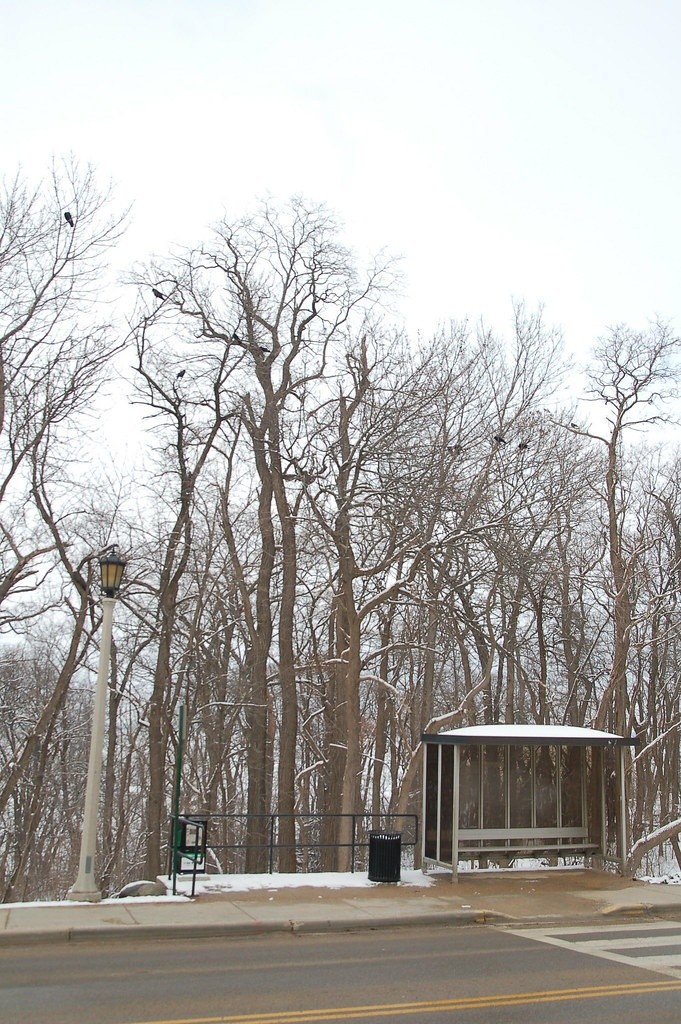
[62,546,127,903]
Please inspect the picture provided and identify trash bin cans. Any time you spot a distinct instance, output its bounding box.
[368,829,402,885]
[172,818,208,875]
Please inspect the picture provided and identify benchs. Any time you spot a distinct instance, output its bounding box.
[455,827,598,872]
